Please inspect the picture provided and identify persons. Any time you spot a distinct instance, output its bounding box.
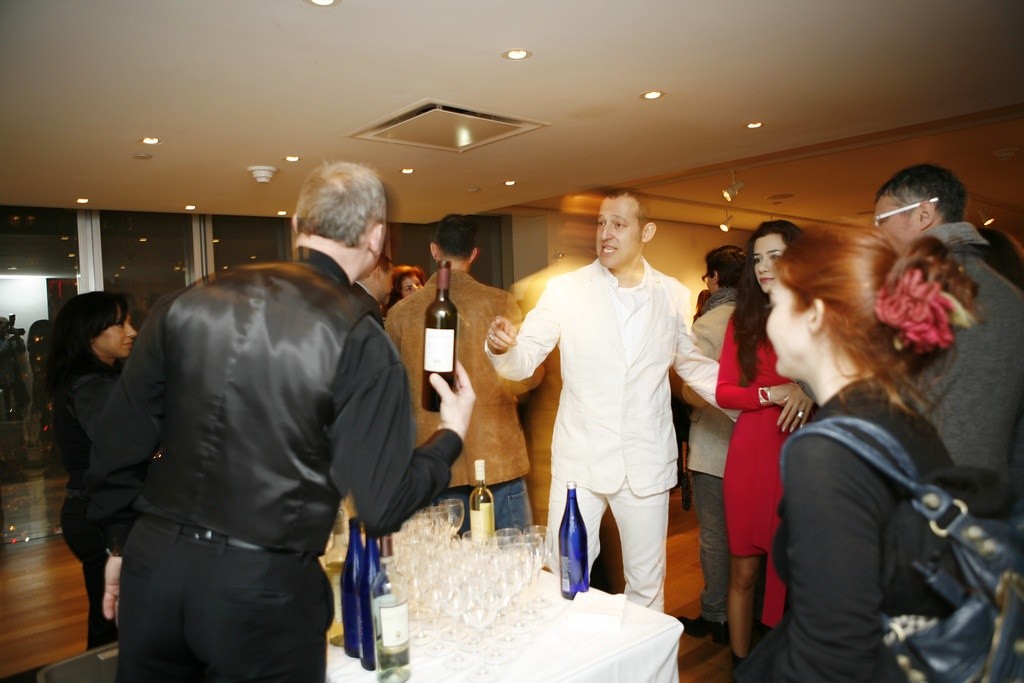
[674,244,741,641]
[715,219,818,667]
[89,159,478,683]
[388,264,426,309]
[352,253,395,330]
[729,227,980,683]
[53,289,139,652]
[1,317,27,421]
[976,227,1024,292]
[872,163,1024,521]
[384,214,546,539]
[487,186,742,611]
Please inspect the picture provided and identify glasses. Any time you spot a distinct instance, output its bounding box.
[874,198,940,226]
[702,273,710,283]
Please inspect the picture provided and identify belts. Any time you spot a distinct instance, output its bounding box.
[179,525,298,554]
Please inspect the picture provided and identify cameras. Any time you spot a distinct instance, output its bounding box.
[4,314,26,335]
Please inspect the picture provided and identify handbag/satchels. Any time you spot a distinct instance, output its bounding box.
[777,419,1024,683]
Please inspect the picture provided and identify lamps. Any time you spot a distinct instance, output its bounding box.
[721,182,745,203]
[719,215,736,232]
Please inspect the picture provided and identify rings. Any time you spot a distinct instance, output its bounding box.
[784,395,789,401]
[797,410,805,418]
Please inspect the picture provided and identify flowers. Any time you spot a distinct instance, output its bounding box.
[871,269,978,354]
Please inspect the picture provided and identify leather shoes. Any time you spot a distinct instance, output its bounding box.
[676,615,728,644]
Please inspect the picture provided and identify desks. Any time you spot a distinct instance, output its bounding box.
[319,529,685,683]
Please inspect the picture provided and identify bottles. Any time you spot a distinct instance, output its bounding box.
[559,482,589,600]
[421,260,458,410]
[471,460,495,543]
[324,509,412,683]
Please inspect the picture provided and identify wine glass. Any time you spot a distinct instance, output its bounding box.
[390,499,554,682]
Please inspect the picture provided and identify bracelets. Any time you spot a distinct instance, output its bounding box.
[757,386,774,406]
